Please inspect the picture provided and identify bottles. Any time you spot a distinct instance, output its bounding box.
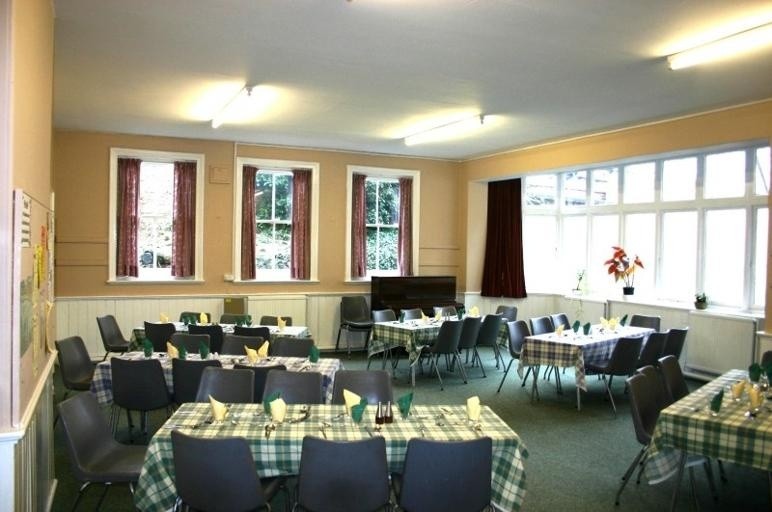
[376,401,385,423]
[385,400,392,422]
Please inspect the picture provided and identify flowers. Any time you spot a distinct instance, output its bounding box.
[604,246,644,287]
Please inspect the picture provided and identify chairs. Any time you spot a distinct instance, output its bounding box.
[180,311,292,325]
[762,349,772,374]
[170,429,292,511]
[110,354,288,440]
[629,315,660,331]
[172,333,314,358]
[96,315,136,361]
[335,297,374,359]
[583,327,688,414]
[293,434,393,510]
[613,354,727,510]
[393,437,492,510]
[197,366,255,402]
[56,391,147,510]
[497,313,571,401]
[263,370,323,404]
[333,370,393,405]
[367,306,469,371]
[144,321,270,353]
[55,335,135,435]
[471,305,517,368]
[407,312,502,392]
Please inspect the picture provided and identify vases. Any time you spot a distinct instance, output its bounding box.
[624,288,634,294]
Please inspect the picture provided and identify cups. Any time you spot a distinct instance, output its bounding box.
[707,373,772,419]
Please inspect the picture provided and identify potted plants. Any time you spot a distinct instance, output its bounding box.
[695,292,707,310]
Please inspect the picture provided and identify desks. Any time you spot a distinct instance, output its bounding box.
[132,320,309,351]
[373,314,508,388]
[99,351,340,436]
[152,402,519,511]
[661,367,772,512]
[523,323,656,411]
